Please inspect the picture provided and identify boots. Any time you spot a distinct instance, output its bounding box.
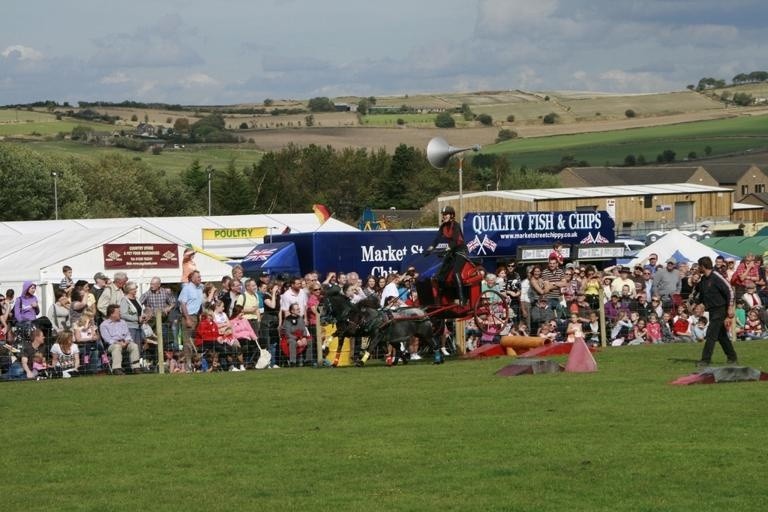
[454,271,468,308]
[436,272,448,305]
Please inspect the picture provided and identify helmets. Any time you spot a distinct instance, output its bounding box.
[441,206,455,217]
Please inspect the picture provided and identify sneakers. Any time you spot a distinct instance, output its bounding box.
[112,368,127,376]
[132,368,146,374]
[83,354,91,364]
[726,358,738,364]
[694,359,712,367]
[99,353,110,365]
[225,362,282,372]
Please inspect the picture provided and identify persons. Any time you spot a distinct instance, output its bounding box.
[424,204,470,308]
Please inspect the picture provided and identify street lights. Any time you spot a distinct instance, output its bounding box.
[49,169,64,219]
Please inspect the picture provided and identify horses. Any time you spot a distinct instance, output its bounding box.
[317,292,392,368]
[347,303,445,367]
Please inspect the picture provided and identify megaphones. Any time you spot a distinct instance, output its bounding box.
[426,137,481,170]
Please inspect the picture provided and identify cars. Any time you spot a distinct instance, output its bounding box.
[613,233,652,252]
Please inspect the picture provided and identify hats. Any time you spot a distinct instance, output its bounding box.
[94,272,110,281]
[665,257,676,264]
[257,270,271,277]
[617,266,633,274]
[611,290,620,298]
[403,274,414,282]
[565,262,575,269]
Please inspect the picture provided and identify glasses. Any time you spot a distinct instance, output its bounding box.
[0,298,6,303]
[507,264,515,268]
[548,323,556,329]
[651,298,660,303]
[565,272,572,277]
[312,287,322,292]
[691,268,698,271]
[644,272,652,276]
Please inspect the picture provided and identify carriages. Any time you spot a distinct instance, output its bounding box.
[314,250,510,368]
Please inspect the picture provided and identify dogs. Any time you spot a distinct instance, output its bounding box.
[507,279,522,292]
